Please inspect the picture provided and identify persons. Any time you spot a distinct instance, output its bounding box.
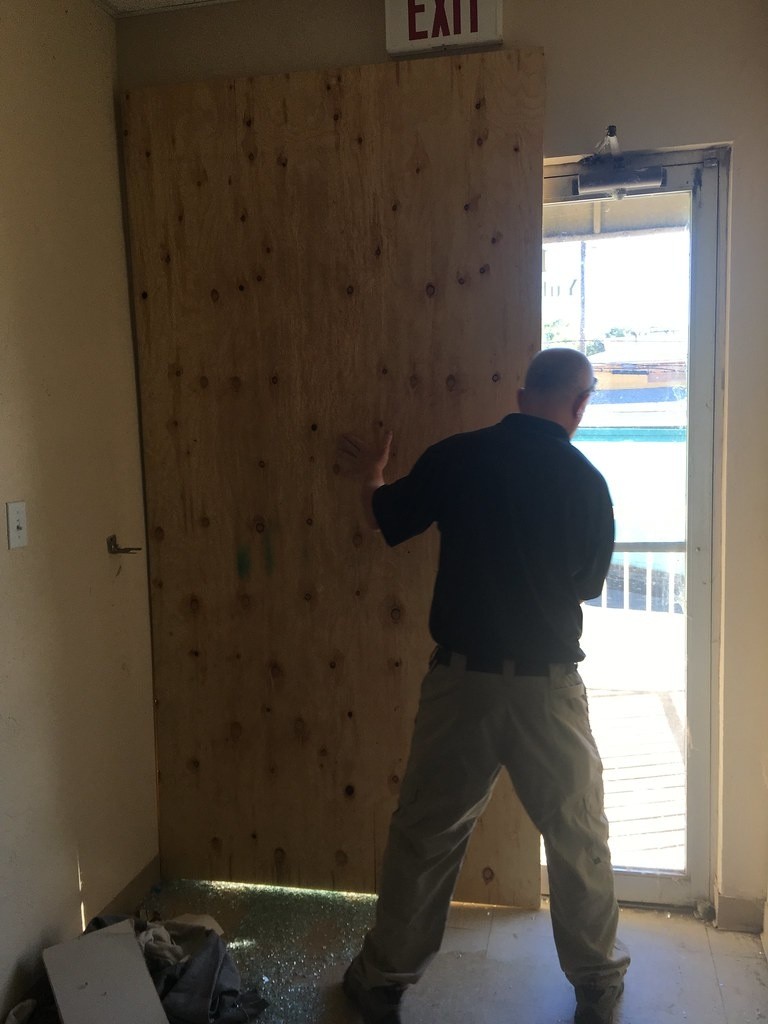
[335,349,632,1024]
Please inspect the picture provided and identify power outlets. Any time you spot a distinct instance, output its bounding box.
[7,500,28,550]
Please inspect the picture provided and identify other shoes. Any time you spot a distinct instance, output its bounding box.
[342,966,404,1024]
[574,981,624,1024]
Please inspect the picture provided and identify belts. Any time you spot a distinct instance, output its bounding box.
[437,648,576,677]
